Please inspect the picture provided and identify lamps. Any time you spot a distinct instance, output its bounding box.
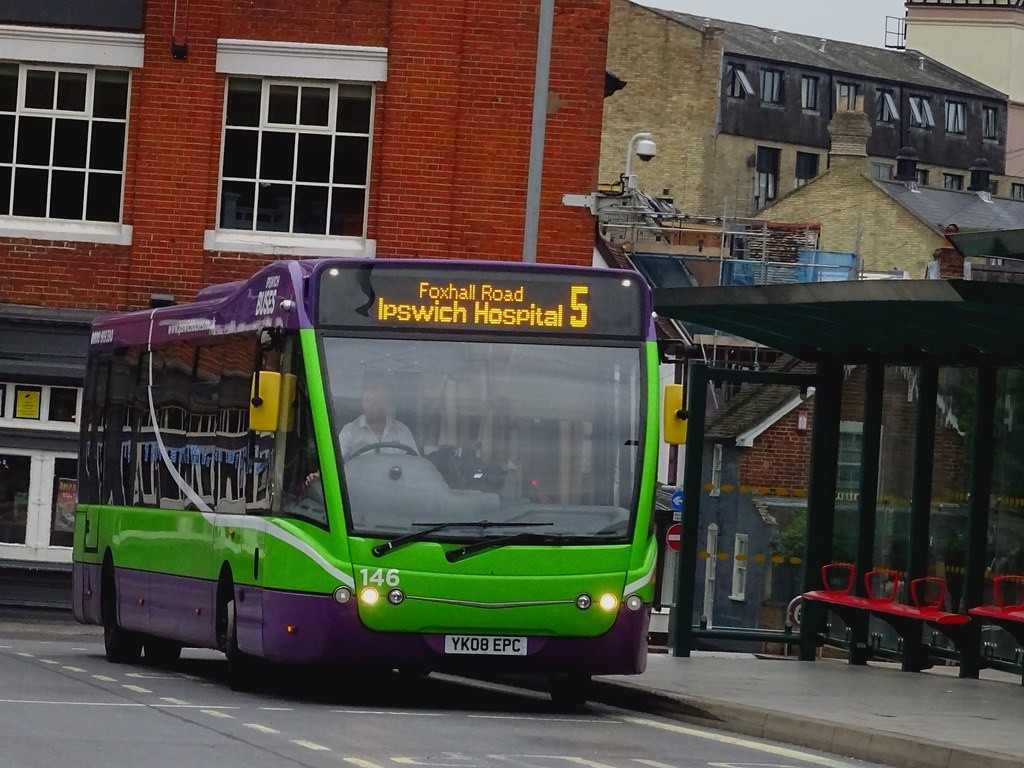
[150,293,174,308]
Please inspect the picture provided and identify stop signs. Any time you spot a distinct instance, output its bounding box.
[666,524,680,553]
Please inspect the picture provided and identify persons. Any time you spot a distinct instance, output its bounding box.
[307,385,421,487]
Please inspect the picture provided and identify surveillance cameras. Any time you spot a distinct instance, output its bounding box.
[636,140,657,162]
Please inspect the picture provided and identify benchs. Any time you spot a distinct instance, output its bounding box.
[969,576,1024,648]
[801,562,971,654]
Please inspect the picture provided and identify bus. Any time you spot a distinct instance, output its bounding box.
[71,252,694,712]
